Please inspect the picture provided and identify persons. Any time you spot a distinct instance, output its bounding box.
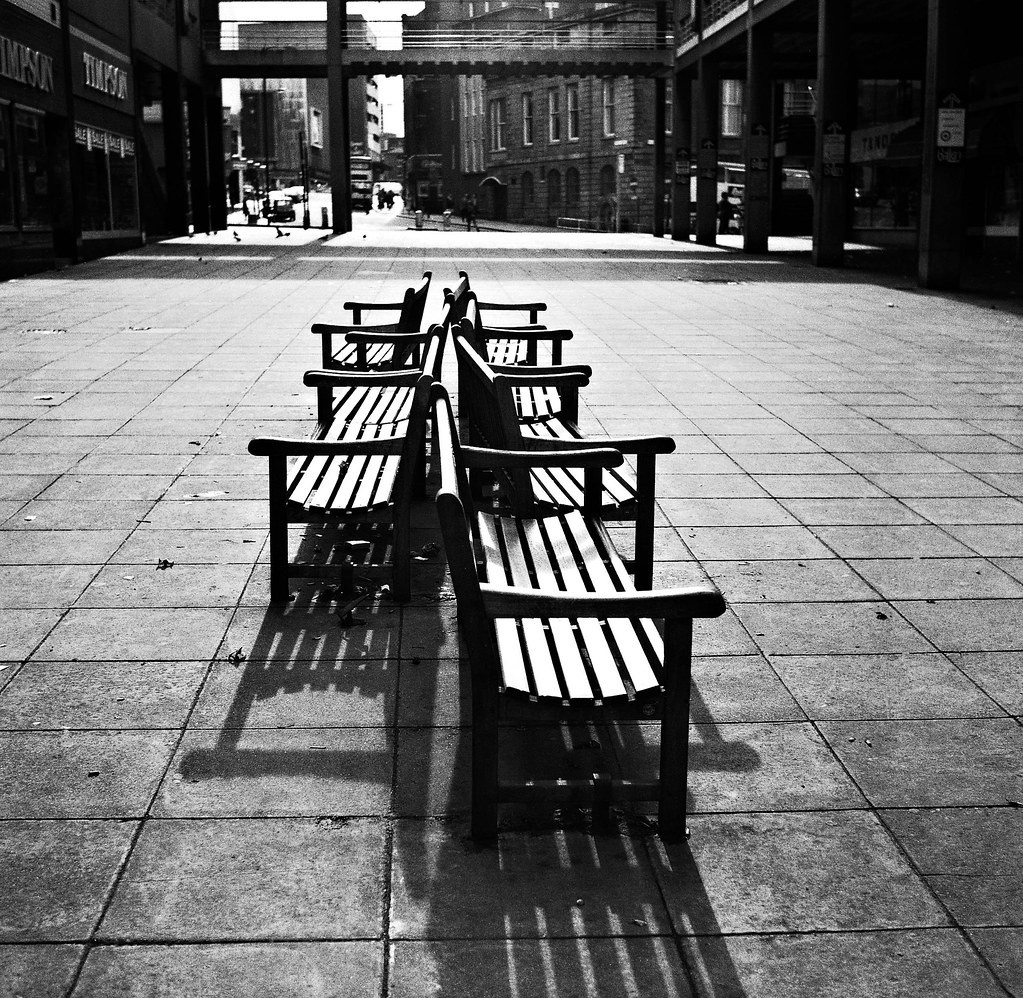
[377,189,395,208]
[717,192,732,234]
[460,193,480,232]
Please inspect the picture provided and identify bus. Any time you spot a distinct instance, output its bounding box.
[684,160,811,234]
[349,153,374,212]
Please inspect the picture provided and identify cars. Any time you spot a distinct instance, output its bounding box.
[269,184,304,221]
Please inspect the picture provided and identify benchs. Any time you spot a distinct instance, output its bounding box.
[247,271,725,849]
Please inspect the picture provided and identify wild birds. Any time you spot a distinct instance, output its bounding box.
[275,227,290,239]
[233,230,241,242]
[363,234,366,238]
